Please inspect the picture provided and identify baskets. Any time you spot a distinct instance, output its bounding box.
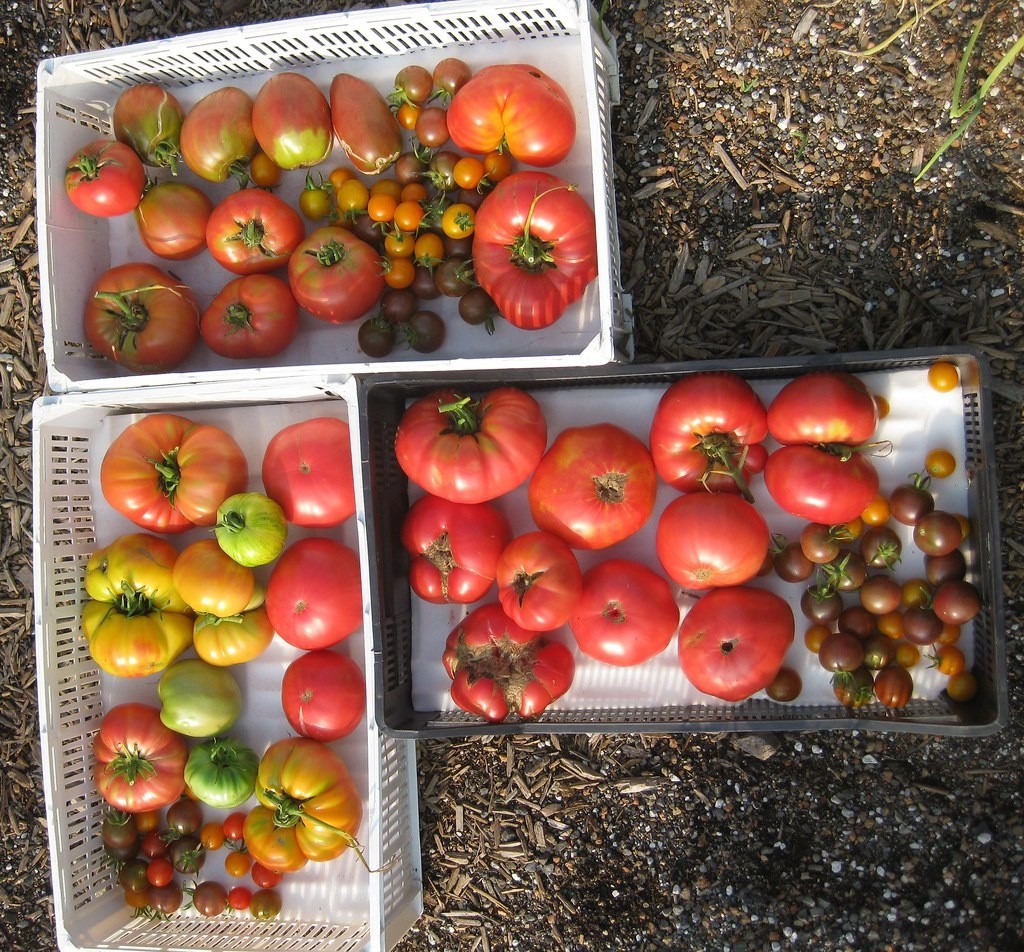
[36,0,632,393]
[31,374,423,952]
[358,342,1008,737]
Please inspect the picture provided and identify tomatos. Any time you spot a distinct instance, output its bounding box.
[79,410,368,925]
[393,360,982,727]
[64,59,597,373]
